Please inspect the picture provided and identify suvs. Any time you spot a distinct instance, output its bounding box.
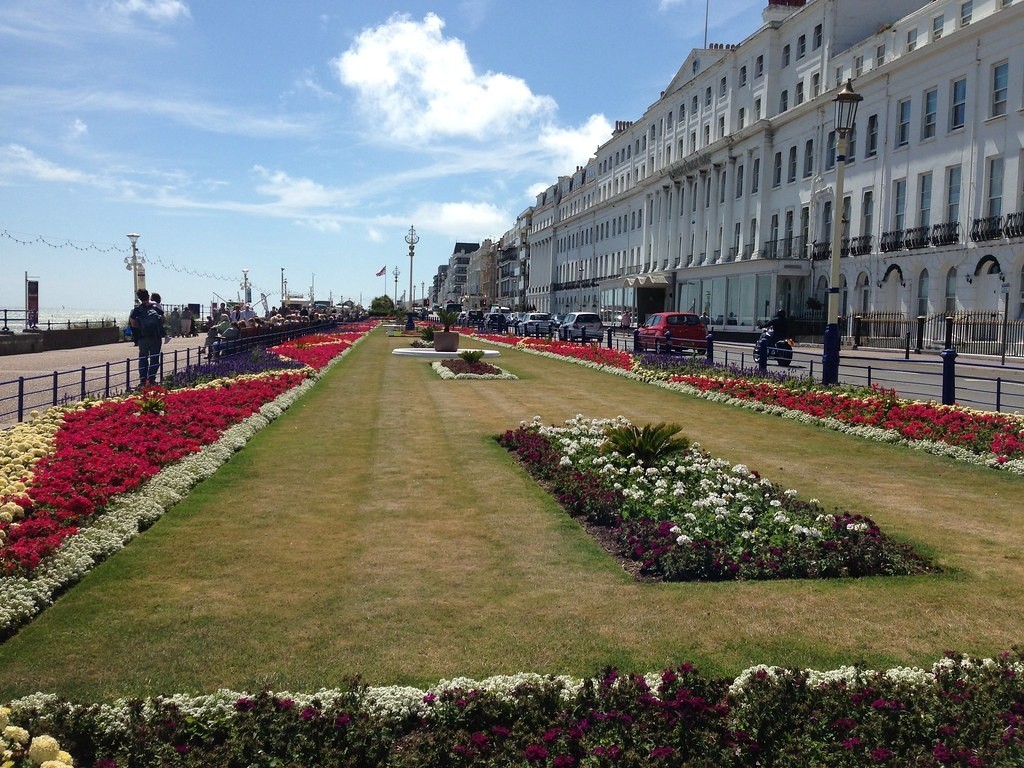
[454,311,524,332]
[517,313,556,337]
[551,313,566,332]
[558,311,604,342]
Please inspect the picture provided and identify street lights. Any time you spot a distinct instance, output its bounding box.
[307,284,315,304]
[328,289,333,307]
[403,224,419,331]
[821,77,864,386]
[392,265,400,310]
[239,268,253,303]
[282,277,290,299]
[124,233,146,307]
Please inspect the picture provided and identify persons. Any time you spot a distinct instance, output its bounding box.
[128,289,171,386]
[621,311,631,337]
[699,312,710,336]
[197,303,274,359]
[759,309,788,347]
[269,302,350,320]
[170,307,195,338]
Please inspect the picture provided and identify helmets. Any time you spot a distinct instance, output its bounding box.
[772,310,786,318]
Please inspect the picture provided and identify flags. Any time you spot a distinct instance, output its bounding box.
[376,266,386,276]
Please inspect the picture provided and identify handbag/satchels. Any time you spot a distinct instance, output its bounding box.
[123,326,132,336]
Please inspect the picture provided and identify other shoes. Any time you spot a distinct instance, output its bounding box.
[628,334,632,337]
[164,337,172,344]
[197,350,205,354]
[204,356,208,359]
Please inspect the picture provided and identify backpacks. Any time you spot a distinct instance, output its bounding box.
[138,304,160,337]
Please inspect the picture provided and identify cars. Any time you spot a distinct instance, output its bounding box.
[637,312,708,355]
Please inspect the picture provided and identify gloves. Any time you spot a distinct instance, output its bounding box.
[757,324,762,329]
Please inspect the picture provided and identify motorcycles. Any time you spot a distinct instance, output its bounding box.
[753,319,794,366]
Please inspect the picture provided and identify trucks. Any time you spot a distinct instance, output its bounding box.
[491,306,510,319]
[443,304,462,314]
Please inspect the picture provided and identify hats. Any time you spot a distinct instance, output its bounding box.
[174,306,179,309]
